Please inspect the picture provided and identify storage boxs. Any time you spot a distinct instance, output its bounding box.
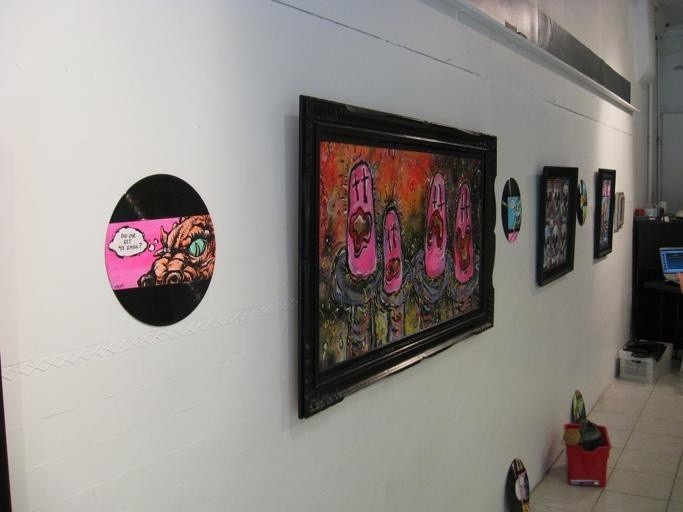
[618,339,672,384]
[563,423,611,487]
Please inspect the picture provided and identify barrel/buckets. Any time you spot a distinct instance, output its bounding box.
[562,420,611,488]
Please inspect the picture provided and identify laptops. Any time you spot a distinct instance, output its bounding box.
[657,245,683,287]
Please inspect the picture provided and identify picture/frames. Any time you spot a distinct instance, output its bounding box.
[535,165,616,287]
[294,94,497,418]
